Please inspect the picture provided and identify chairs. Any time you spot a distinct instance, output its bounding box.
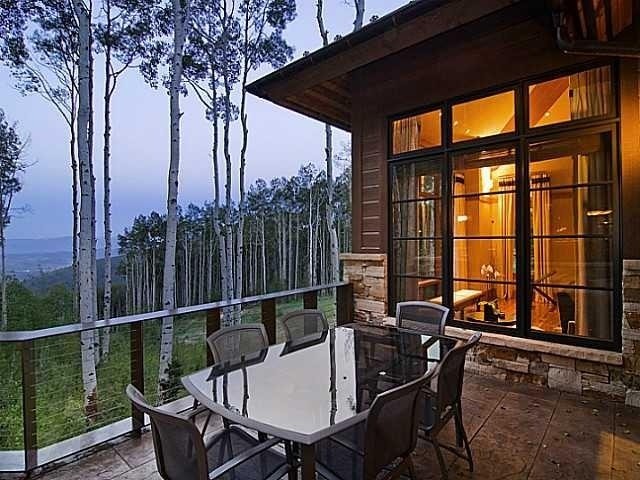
[206,324,268,362]
[283,310,328,340]
[417,335,481,476]
[395,301,449,334]
[128,385,301,480]
[315,369,433,480]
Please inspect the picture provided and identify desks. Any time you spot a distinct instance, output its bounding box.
[182,324,461,480]
